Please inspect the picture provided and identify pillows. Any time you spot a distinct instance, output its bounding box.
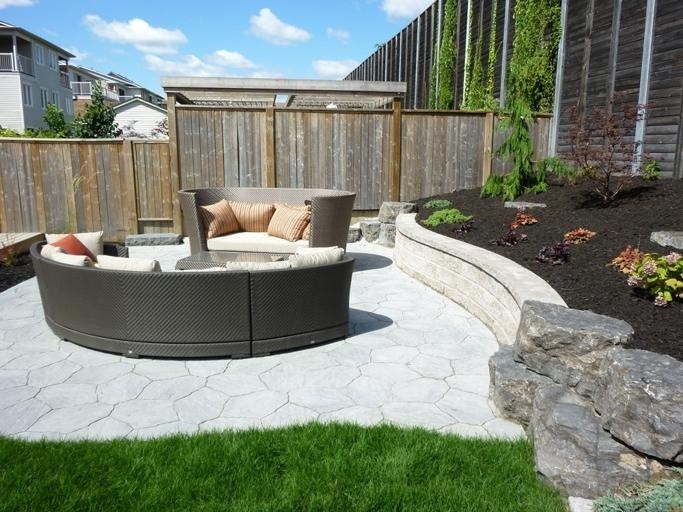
[40,227,162,273]
[29,227,358,357]
[224,245,347,271]
[267,203,311,242]
[230,197,274,234]
[197,197,241,238]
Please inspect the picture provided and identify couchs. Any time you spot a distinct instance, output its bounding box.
[175,188,357,264]
